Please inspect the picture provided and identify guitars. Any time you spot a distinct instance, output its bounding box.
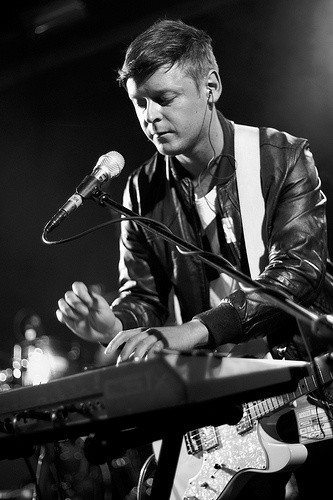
[153,351,333,500]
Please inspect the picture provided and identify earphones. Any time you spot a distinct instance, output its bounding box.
[206,87,213,98]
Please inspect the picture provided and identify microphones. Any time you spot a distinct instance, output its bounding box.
[45,149,125,234]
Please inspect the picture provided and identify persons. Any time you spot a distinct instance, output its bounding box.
[54,20,333,499]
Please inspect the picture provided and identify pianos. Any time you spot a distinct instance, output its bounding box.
[1,351,314,460]
